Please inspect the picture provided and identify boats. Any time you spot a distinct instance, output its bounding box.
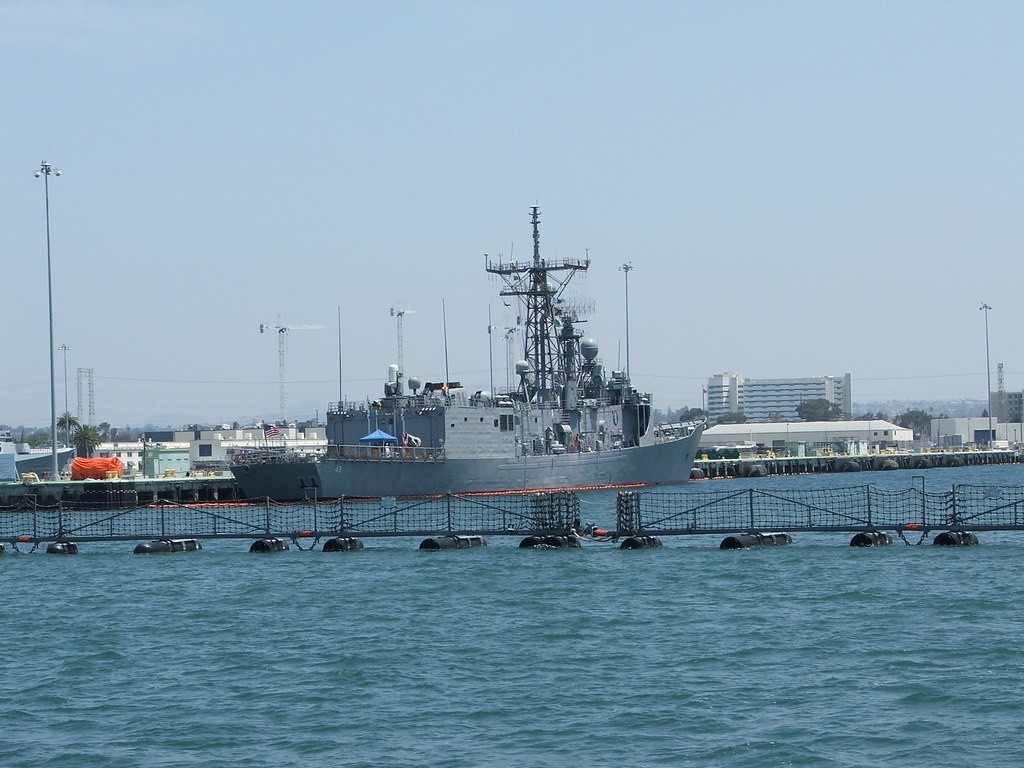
[227,418,320,506]
[314,196,708,499]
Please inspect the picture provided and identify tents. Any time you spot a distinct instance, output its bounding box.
[359,429,398,458]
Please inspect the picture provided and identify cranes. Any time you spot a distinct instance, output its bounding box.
[256,320,327,422]
[389,307,419,385]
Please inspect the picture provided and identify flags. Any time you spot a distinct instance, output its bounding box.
[264,424,281,438]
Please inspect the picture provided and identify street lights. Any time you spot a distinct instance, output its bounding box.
[617,259,635,380]
[34,160,65,482]
[58,343,71,447]
[980,301,995,451]
[137,437,153,480]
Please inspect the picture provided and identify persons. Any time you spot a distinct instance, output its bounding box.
[570,436,580,452]
